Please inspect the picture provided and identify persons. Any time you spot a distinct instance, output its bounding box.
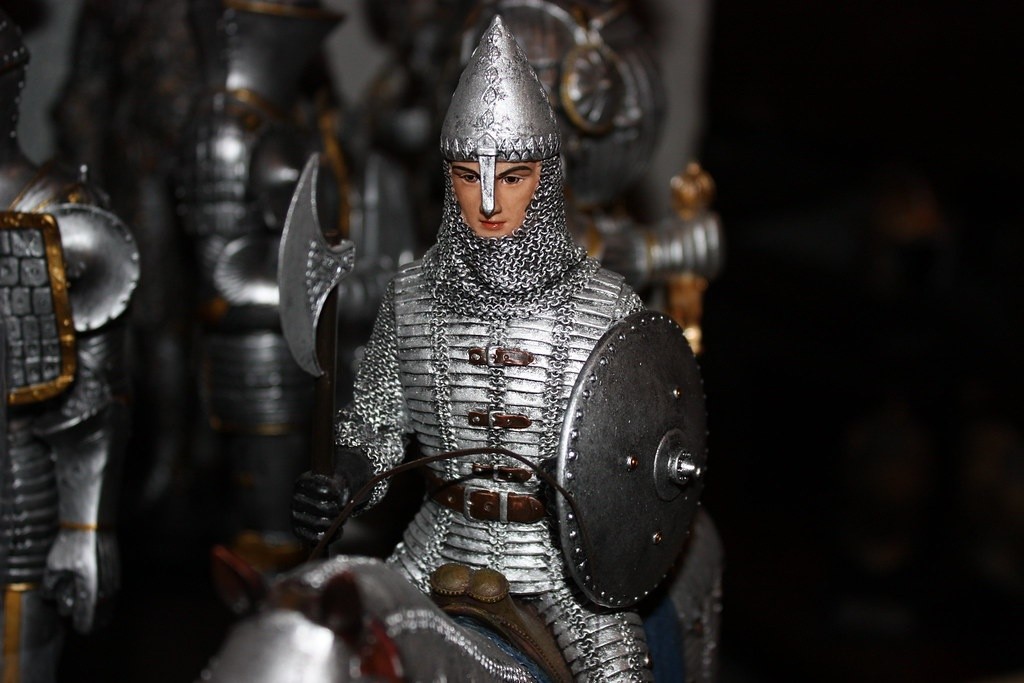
[0,0,719,683]
[282,14,711,683]
